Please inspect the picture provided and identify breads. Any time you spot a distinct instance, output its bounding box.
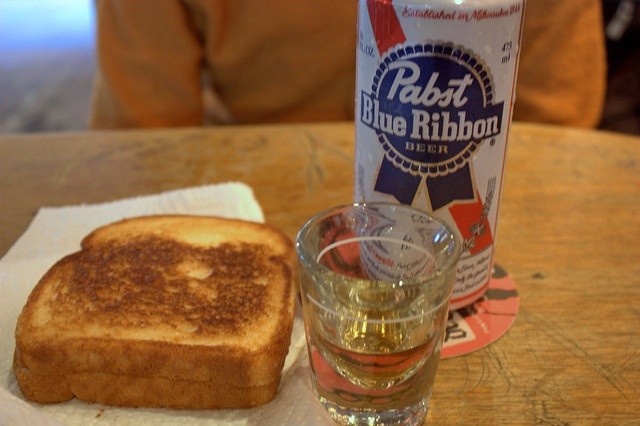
[12,214,298,411]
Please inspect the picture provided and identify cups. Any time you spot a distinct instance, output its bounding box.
[296,201,462,426]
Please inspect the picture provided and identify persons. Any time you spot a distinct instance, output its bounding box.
[83,0,608,131]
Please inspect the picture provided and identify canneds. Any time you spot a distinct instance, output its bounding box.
[356,1,526,312]
